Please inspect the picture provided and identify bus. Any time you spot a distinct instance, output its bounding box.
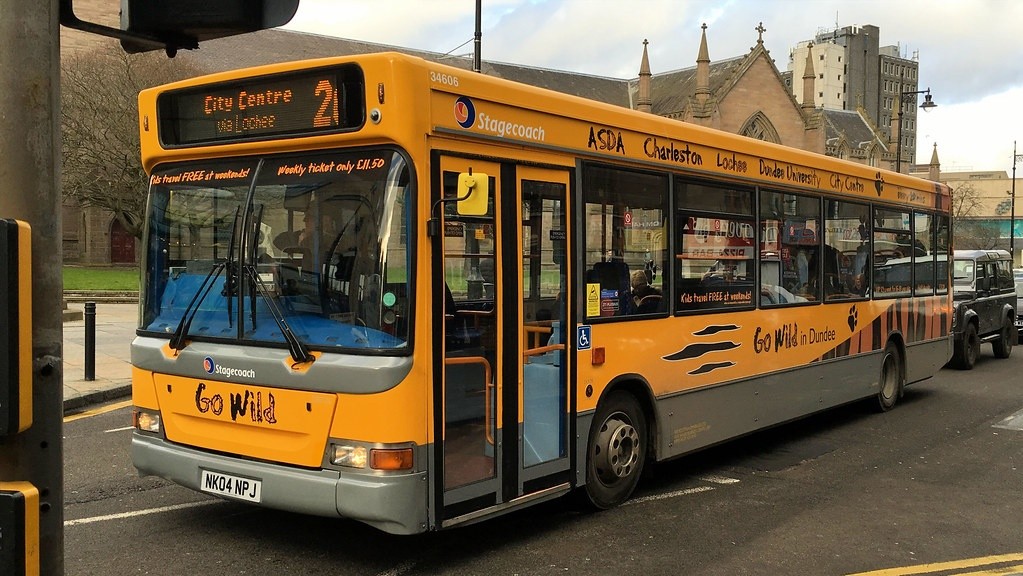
[130,52,954,537]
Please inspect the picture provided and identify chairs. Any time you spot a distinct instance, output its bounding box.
[635,267,910,315]
[445,279,492,347]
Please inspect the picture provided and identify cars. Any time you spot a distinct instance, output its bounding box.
[1012,268,1023,336]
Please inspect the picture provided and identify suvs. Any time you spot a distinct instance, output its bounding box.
[883,250,1017,370]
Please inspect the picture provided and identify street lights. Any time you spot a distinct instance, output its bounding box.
[896,88,938,173]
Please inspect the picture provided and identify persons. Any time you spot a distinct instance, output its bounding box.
[727,223,749,246]
[271,199,372,288]
[480,250,495,300]
[797,229,852,267]
[626,270,662,315]
[893,229,927,259]
